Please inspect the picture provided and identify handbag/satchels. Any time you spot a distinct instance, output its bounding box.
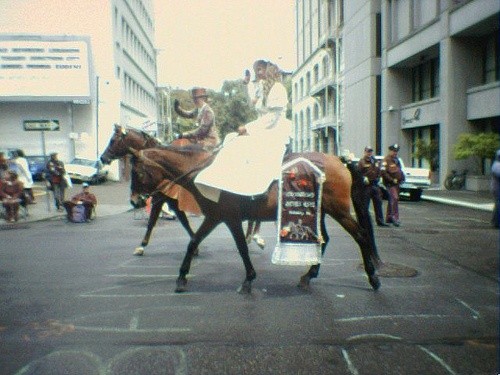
[63,174,72,188]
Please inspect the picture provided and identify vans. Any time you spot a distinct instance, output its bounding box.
[0,148,24,168]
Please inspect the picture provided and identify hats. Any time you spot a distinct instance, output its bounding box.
[82,181,89,187]
[191,88,207,98]
[388,143,401,151]
[364,146,373,152]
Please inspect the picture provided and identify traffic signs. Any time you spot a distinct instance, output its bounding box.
[23,120,60,132]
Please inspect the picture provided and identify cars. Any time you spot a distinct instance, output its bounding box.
[65,156,107,184]
[8,155,63,180]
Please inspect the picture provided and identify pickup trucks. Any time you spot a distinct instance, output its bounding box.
[353,156,431,202]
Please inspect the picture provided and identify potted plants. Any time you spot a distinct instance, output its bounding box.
[452,130,500,192]
[412,137,440,183]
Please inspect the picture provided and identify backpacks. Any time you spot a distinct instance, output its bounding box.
[70,205,87,223]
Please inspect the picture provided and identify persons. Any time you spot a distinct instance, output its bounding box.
[43,152,69,213]
[1,151,13,195]
[384,142,406,228]
[356,148,390,229]
[193,62,291,214]
[243,59,271,119]
[489,149,499,230]
[169,88,222,153]
[10,149,38,206]
[0,171,23,223]
[62,181,96,223]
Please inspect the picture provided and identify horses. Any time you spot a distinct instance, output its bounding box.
[99,123,267,256]
[129,130,386,296]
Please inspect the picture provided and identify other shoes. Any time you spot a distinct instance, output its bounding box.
[377,222,389,226]
[386,219,394,223]
[393,222,400,227]
